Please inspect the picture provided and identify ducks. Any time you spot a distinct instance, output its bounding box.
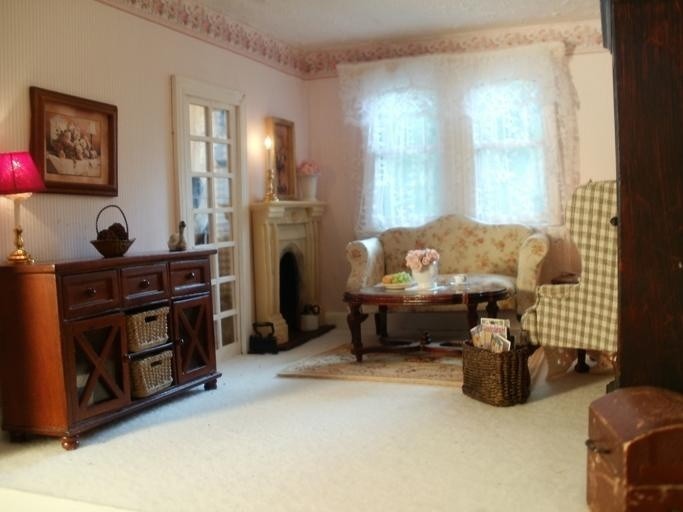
[167,220,187,253]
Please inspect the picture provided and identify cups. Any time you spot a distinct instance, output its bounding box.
[453,272,469,283]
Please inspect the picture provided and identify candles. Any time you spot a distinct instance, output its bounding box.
[264,136,273,169]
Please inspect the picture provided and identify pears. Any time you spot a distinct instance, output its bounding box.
[382,276,393,283]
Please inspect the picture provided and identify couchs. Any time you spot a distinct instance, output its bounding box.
[345,215,551,323]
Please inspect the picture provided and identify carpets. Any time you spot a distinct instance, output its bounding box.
[276,332,547,389]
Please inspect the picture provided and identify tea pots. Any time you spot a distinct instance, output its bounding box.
[299,303,321,332]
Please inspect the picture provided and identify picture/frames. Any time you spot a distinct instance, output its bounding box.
[265,115,298,200]
[29,85,118,197]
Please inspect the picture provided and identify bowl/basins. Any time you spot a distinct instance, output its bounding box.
[89,237,136,259]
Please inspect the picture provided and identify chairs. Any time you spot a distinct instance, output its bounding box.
[519,179,619,382]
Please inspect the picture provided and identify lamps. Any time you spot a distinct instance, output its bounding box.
[1,150,48,263]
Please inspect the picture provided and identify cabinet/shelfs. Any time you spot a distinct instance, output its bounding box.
[0,249,222,450]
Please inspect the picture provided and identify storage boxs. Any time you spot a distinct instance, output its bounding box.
[585,385,683,512]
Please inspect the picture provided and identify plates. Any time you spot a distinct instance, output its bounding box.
[374,281,418,289]
[450,281,468,286]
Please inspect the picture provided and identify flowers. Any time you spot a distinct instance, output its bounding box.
[298,159,321,175]
[405,247,440,273]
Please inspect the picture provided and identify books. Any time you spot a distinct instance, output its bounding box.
[469,316,515,353]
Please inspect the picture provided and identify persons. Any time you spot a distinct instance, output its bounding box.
[53,120,98,162]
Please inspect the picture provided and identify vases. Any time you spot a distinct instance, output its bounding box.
[301,173,318,201]
[411,261,437,283]
